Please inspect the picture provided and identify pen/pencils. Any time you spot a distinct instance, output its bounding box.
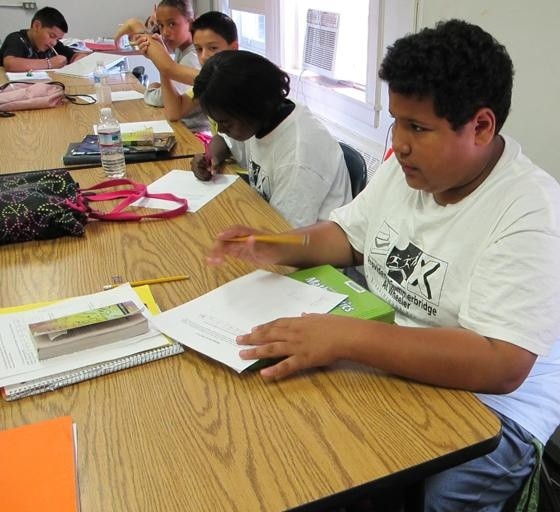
[204,141,215,183]
[51,45,59,56]
[214,234,311,246]
[103,275,190,290]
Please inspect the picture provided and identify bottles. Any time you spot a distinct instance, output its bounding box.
[93,61,113,108]
[95,108,126,182]
[117,22,129,49]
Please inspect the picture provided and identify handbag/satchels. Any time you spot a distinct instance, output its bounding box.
[0,170,86,243]
[0,81,65,111]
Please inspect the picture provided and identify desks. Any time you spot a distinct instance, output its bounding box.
[0,59,148,93]
[0,83,209,175]
[0,155,503,511]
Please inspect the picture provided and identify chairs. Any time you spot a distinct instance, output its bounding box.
[338,140,369,202]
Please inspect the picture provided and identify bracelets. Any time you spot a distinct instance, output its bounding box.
[46,57,52,70]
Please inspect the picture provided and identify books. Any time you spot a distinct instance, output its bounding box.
[1,264,393,400]
[1,416,79,512]
[63,39,93,53]
[62,119,175,166]
[4,72,51,81]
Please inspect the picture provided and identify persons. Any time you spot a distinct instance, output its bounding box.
[0,7,87,73]
[205,17,560,510]
[143,1,203,107]
[191,49,352,228]
[113,11,239,135]
